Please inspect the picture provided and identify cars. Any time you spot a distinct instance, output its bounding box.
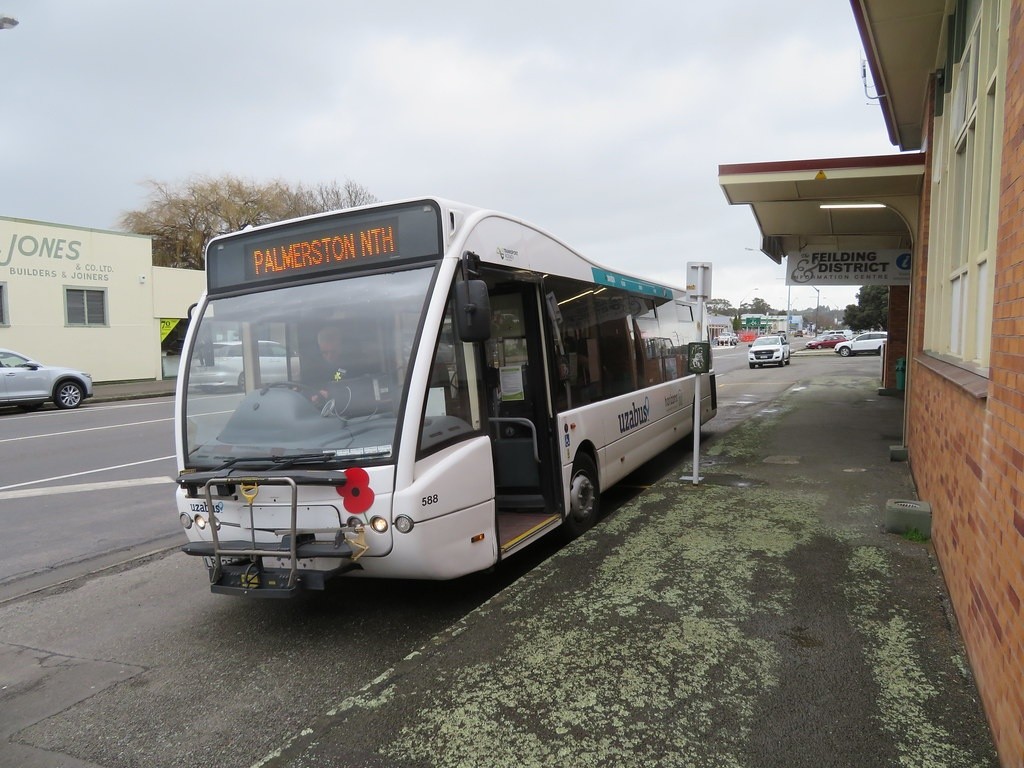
[713,331,738,346]
[0,348,93,411]
[769,327,852,350]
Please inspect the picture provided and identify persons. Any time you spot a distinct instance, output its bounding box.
[304,324,381,419]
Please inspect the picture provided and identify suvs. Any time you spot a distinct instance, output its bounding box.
[747,335,790,369]
[833,331,888,357]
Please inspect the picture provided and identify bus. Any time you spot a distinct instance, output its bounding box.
[173,196,719,602]
